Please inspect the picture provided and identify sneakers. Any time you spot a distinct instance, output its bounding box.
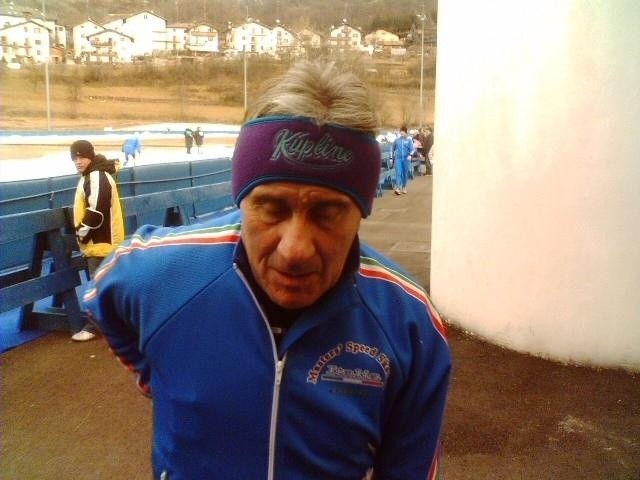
[395,188,401,195]
[402,187,407,194]
[71,330,96,342]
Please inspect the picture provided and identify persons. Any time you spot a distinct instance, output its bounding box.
[75,64,452,479]
[193,125,205,154]
[374,116,439,201]
[122,132,142,163]
[65,139,128,345]
[183,127,194,154]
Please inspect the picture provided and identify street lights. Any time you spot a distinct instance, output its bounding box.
[415,13,424,128]
[240,5,251,111]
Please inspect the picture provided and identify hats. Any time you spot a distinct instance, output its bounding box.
[70,140,95,161]
